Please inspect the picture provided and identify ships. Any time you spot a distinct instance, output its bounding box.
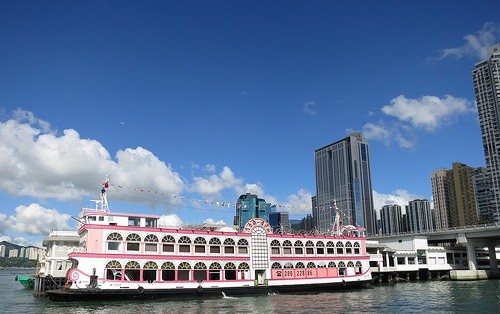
[45,172,375,299]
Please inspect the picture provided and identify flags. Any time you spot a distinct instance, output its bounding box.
[101,187,105,194]
[111,184,363,228]
[105,181,109,188]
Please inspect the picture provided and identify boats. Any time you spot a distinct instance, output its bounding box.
[34,229,499,284]
[12,273,35,290]
